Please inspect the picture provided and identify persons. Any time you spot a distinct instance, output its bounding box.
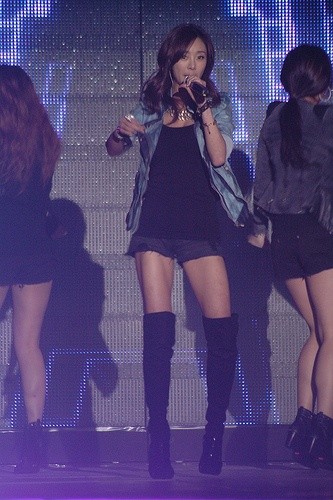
[106,22,254,479]
[0,65,63,477]
[246,43,333,470]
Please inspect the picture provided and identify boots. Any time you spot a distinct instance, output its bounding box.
[199,313,238,475]
[143,311,176,480]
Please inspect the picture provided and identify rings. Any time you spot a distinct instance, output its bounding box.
[125,114,136,122]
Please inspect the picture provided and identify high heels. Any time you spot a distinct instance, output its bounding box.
[287,407,316,465]
[307,413,333,470]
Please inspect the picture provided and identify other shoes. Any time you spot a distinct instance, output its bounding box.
[15,419,45,473]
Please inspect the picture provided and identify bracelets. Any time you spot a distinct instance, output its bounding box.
[114,126,123,142]
[197,100,213,117]
[202,120,217,130]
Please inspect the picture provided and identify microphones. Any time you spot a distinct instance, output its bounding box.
[184,76,209,97]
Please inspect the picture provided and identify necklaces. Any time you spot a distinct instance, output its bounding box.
[167,100,193,121]
